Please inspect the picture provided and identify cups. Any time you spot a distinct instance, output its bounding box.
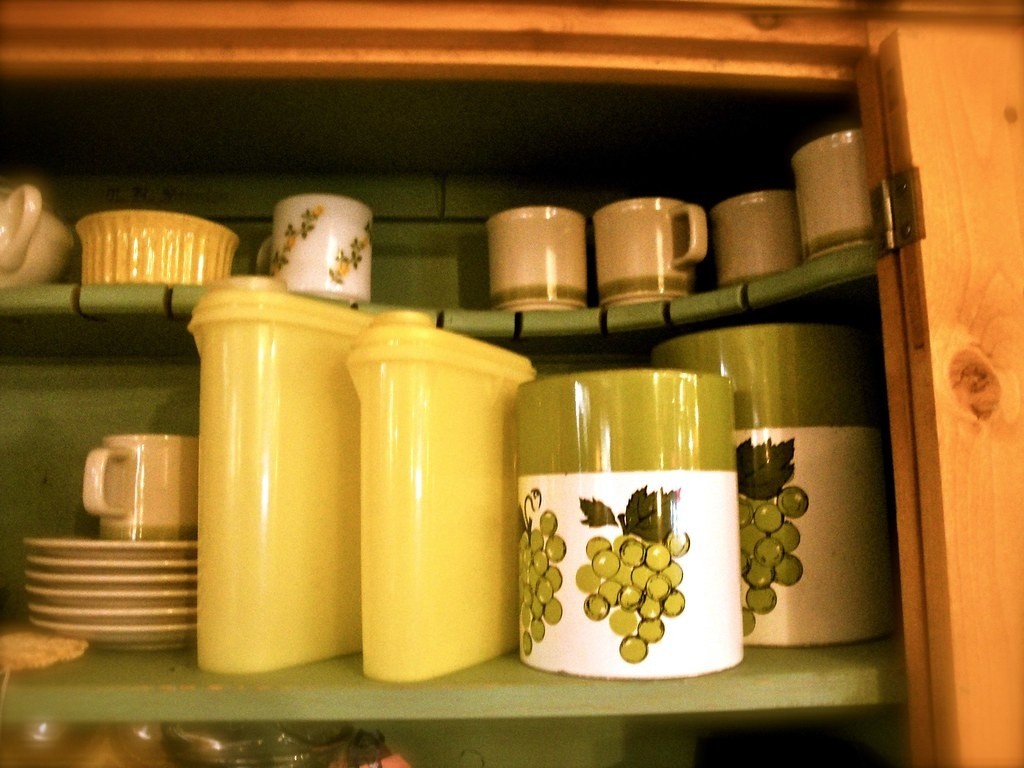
[594,197,706,308]
[82,434,198,539]
[1,184,67,288]
[489,206,587,313]
[514,369,744,680]
[255,194,372,300]
[792,128,877,261]
[711,189,799,285]
[653,323,894,646]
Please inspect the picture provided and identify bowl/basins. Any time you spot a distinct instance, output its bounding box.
[73,210,240,286]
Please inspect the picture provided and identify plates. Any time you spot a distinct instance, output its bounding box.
[25,536,198,558]
[24,581,197,605]
[24,567,197,589]
[25,553,197,573]
[27,599,197,624]
[27,617,199,650]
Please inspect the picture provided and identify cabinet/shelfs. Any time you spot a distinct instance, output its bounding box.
[0,0,1024,768]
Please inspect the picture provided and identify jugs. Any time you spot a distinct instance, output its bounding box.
[187,274,375,673]
[350,309,537,683]
